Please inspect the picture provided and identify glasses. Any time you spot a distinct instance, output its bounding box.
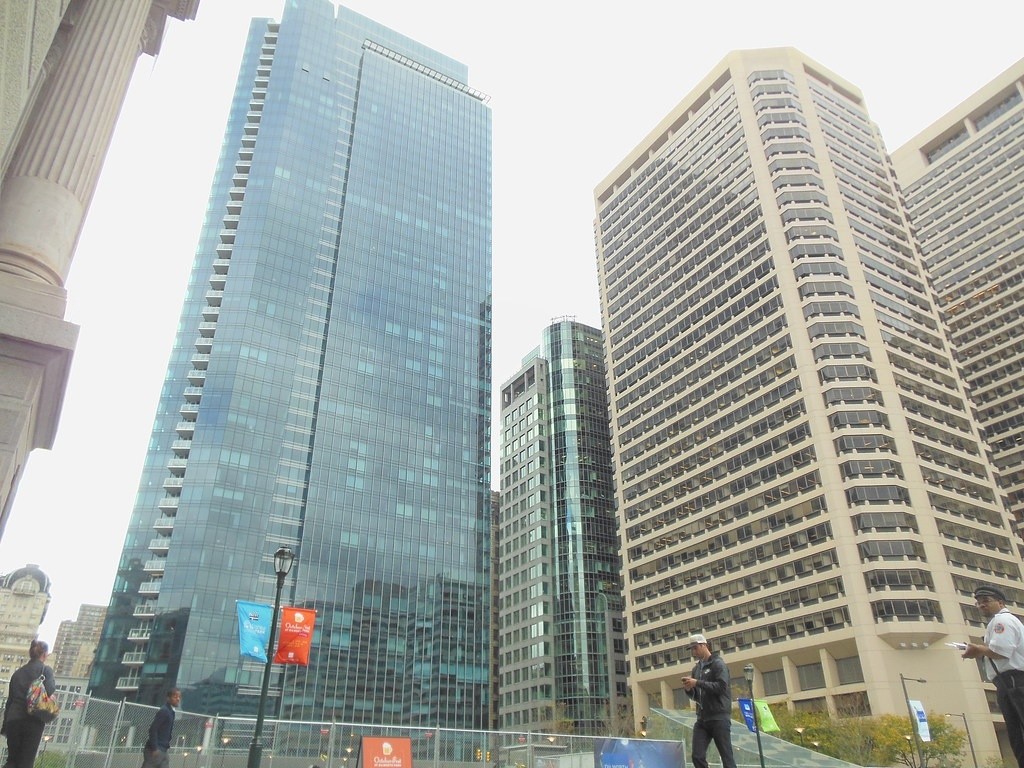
[974,599,999,607]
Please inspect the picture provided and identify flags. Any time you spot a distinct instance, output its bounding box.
[236,600,275,663]
[910,701,931,742]
[73,699,87,708]
[274,606,316,665]
[754,699,781,733]
[737,698,759,732]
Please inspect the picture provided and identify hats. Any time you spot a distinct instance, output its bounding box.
[974,586,1005,601]
[685,634,707,651]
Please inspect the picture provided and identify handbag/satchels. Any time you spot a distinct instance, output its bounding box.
[26,664,58,722]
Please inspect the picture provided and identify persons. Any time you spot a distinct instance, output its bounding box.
[0,639,60,768]
[141,688,181,768]
[680,634,738,768]
[944,586,1024,768]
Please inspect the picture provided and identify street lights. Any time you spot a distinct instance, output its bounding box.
[246,546,296,768]
[945,712,977,767]
[899,672,928,768]
[743,664,768,768]
